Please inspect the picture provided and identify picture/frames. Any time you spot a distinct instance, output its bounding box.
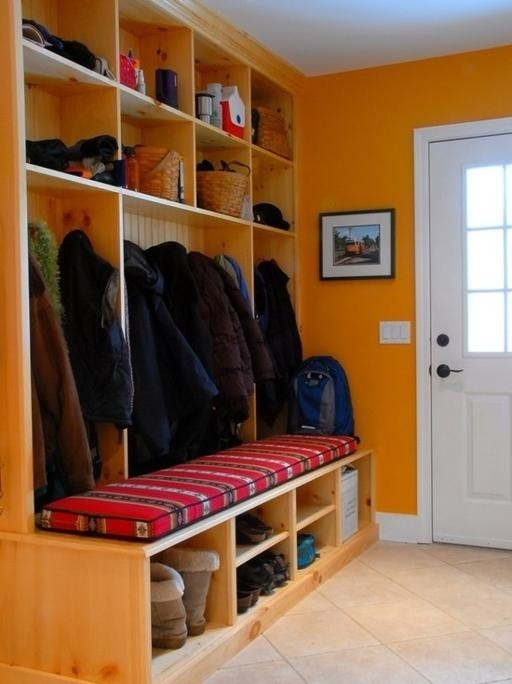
[318,208,395,281]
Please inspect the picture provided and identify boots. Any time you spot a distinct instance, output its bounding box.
[150,548,220,648]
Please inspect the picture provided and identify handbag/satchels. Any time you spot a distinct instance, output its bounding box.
[239,550,288,594]
[298,534,320,569]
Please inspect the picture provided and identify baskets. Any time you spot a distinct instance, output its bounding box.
[258,107,289,158]
[120,54,136,89]
[135,146,180,200]
[196,161,250,217]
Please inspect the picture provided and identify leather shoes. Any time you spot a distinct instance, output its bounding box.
[235,585,261,613]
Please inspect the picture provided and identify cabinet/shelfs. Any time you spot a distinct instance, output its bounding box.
[1,0,380,683]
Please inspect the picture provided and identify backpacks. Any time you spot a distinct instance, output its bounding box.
[288,356,354,436]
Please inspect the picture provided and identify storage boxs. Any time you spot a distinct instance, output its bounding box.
[340,463,360,543]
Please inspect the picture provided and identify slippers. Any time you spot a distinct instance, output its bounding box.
[237,514,274,544]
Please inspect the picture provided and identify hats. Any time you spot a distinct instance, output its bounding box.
[253,204,289,231]
